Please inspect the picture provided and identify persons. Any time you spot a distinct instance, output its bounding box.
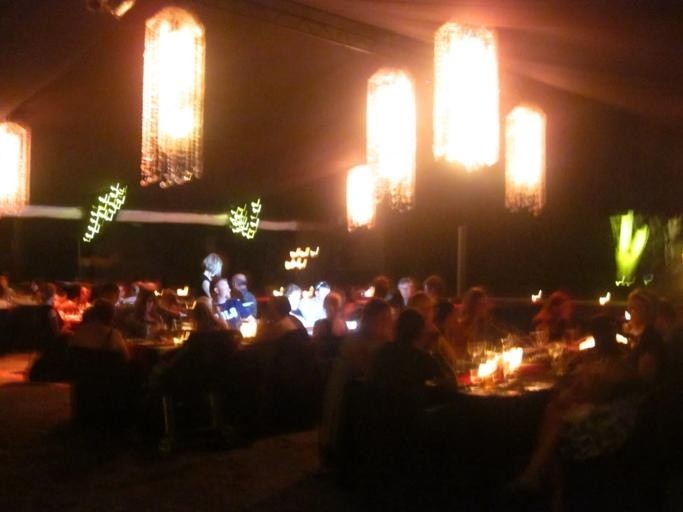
[2,273,683,511]
[193,253,223,303]
